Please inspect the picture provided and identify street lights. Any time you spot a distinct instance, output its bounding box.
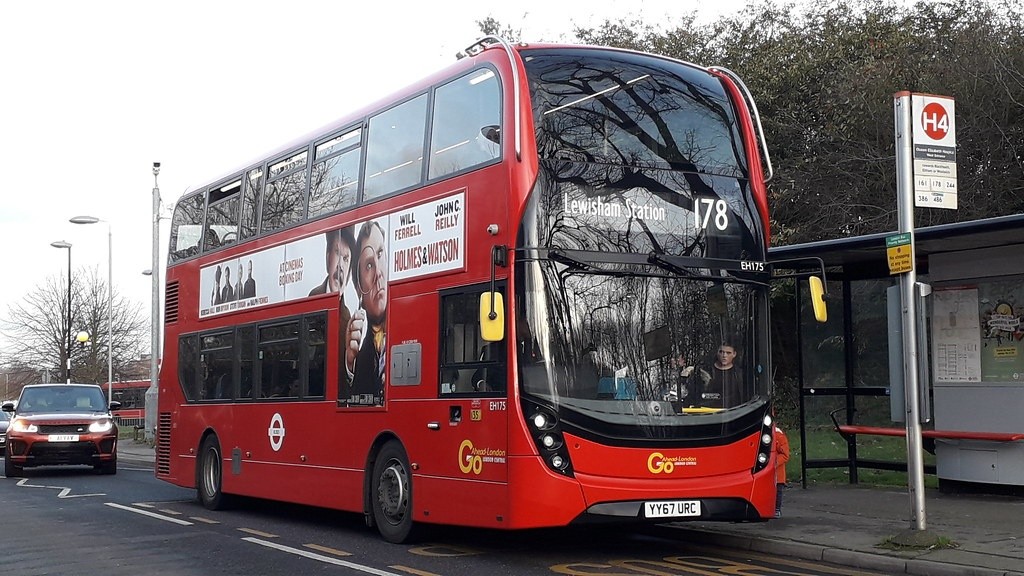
[51,240,73,386]
[69,215,113,420]
[148,161,164,446]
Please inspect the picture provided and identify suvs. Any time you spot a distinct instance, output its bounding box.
[0,381,122,475]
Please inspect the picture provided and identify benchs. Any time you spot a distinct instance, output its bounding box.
[831,406,1024,457]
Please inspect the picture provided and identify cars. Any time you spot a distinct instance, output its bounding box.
[0,407,12,456]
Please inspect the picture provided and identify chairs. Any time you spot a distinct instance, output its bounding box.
[202,366,323,398]
[36,395,47,407]
[76,396,93,409]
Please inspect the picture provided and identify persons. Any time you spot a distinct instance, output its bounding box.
[309,222,386,406]
[471,308,545,393]
[212,261,256,306]
[662,342,746,415]
[288,377,300,397]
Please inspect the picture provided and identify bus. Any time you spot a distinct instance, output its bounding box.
[153,33,828,548]
[101,380,151,429]
